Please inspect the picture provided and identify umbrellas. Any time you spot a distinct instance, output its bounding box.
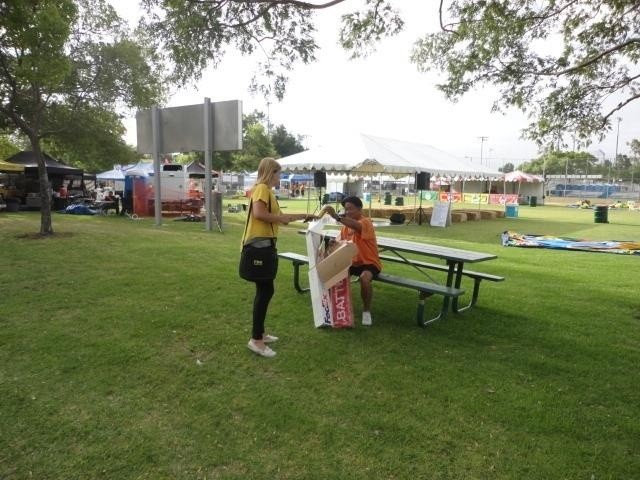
[504,170,534,195]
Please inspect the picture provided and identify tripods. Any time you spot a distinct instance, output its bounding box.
[313,187,323,215]
[407,189,431,226]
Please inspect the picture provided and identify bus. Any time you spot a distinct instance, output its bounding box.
[0,162,27,211]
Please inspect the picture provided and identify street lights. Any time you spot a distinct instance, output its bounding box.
[266,101,273,138]
[612,115,624,181]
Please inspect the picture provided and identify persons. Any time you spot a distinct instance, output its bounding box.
[101,191,117,214]
[322,196,383,326]
[239,157,318,358]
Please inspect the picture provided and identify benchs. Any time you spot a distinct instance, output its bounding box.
[276,251,505,328]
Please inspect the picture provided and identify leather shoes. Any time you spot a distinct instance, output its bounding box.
[248,334,278,357]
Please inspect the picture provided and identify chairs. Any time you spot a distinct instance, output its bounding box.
[105,198,119,214]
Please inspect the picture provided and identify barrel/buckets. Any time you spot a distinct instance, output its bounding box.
[385,192,392,204]
[60,188,68,198]
[529,195,537,206]
[594,205,608,223]
[505,204,519,217]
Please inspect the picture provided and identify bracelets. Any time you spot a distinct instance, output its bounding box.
[336,215,341,221]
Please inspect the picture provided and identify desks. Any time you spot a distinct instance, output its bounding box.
[298,229,497,317]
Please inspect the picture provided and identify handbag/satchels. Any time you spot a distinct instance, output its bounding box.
[239,245,278,281]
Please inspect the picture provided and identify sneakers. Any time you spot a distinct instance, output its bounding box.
[362,311,371,325]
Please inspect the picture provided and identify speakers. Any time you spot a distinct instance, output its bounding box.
[390,214,405,224]
[417,171,430,190]
[314,172,326,187]
